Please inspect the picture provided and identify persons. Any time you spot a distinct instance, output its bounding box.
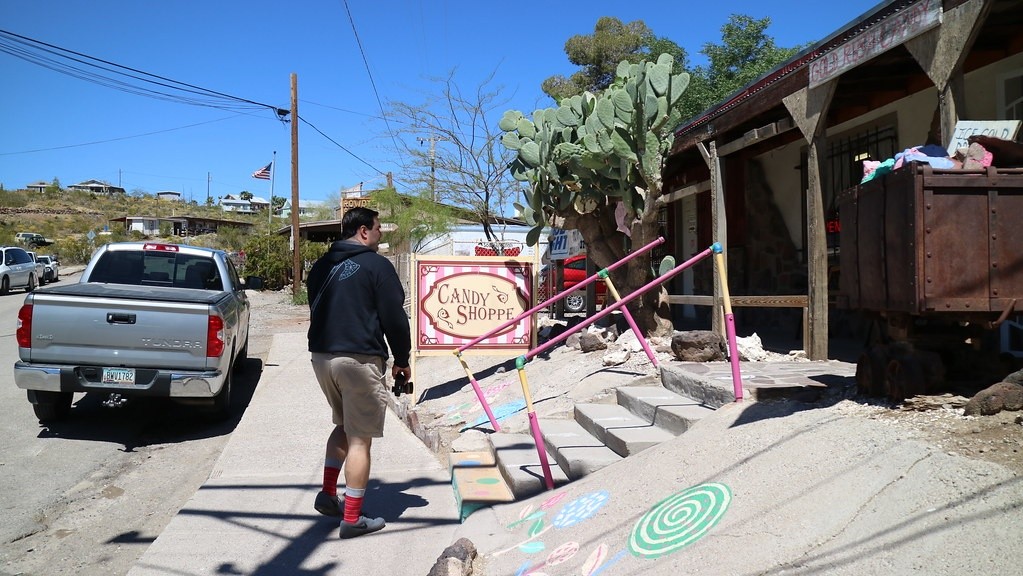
[51,252,59,262]
[307,206,412,538]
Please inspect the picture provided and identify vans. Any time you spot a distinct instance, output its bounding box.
[14,232,45,245]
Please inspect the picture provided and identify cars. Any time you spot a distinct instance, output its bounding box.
[537,253,610,312]
[26,252,59,285]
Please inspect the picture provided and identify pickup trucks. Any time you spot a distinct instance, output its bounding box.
[14,241,264,424]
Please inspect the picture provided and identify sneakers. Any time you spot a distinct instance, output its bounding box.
[314,491,345,517]
[340,516,385,539]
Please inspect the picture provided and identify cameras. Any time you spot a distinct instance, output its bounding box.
[391,374,414,398]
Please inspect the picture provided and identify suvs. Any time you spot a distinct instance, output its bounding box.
[0,246,35,296]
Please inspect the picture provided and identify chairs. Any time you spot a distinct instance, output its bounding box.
[194,262,216,290]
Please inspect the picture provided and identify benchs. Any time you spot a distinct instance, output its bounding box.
[185,265,211,290]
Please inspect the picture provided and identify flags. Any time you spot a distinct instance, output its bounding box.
[253,161,272,179]
